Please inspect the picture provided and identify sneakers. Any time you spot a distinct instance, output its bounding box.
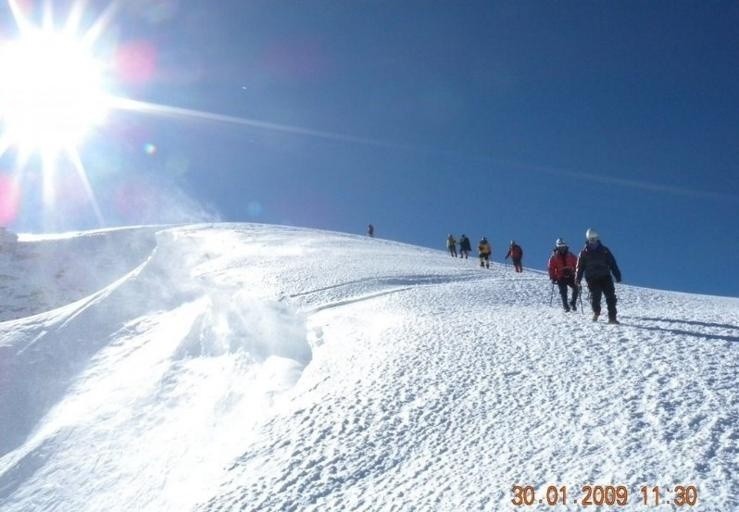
[592,312,600,321]
[570,302,576,310]
[565,306,570,313]
[608,319,619,324]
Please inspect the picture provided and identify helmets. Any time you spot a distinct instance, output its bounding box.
[510,240,515,245]
[586,228,600,238]
[556,238,566,247]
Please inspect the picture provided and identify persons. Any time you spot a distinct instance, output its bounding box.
[574,228,621,323]
[447,233,457,257]
[478,235,491,268]
[367,223,374,237]
[548,238,578,312]
[459,234,471,259]
[505,240,523,273]
